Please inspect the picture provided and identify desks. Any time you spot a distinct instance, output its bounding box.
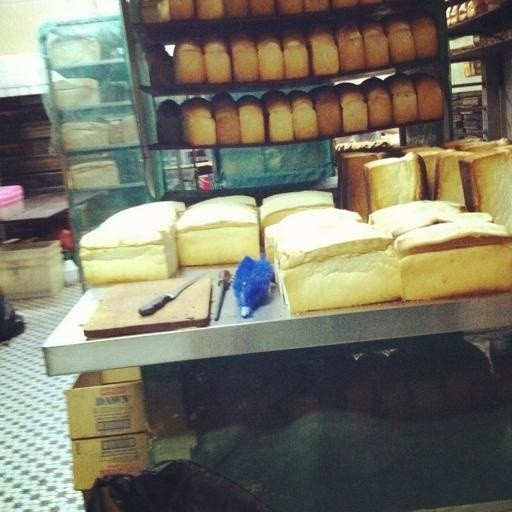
[40,262,511,382]
[1,190,109,224]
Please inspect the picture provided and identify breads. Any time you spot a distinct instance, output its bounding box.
[79,139,511,315]
[48,32,139,193]
[127,0,446,146]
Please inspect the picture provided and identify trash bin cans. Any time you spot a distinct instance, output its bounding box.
[87,460,277,512]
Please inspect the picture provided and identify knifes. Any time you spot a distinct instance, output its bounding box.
[139,269,211,317]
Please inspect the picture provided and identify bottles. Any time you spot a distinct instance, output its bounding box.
[196,150,213,192]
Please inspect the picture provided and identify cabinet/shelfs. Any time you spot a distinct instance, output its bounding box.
[444,0,511,64]
[43,16,171,288]
[114,6,454,201]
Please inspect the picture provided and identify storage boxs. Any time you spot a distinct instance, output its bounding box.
[67,432,148,493]
[61,364,149,439]
[1,239,65,300]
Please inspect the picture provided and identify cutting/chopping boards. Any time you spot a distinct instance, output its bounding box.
[84,278,210,338]
